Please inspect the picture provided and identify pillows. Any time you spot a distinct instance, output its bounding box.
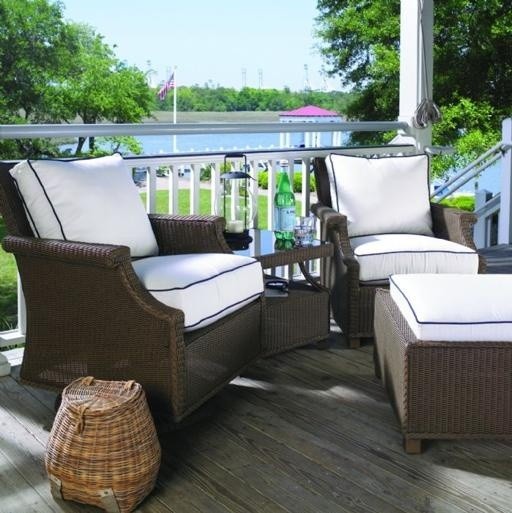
[324,153,437,237]
[7,150,161,258]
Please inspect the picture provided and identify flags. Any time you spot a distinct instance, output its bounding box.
[158,72,174,101]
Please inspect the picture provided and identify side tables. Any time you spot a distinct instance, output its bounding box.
[225,226,334,359]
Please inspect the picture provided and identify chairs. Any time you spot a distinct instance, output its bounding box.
[1,154,268,424]
[370,271,512,456]
[308,154,487,349]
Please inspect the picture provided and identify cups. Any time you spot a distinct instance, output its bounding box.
[296,215,316,249]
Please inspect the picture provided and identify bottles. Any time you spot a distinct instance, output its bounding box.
[273,159,297,251]
